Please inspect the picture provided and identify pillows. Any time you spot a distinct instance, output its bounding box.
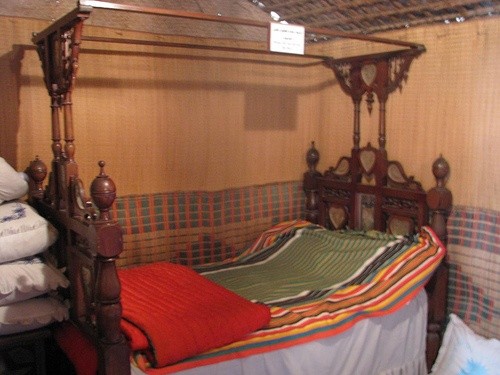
[0,156,32,206]
[427,312,500,375]
[0,293,71,337]
[0,199,59,265]
[0,253,71,308]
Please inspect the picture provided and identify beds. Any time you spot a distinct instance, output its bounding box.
[83,218,450,375]
